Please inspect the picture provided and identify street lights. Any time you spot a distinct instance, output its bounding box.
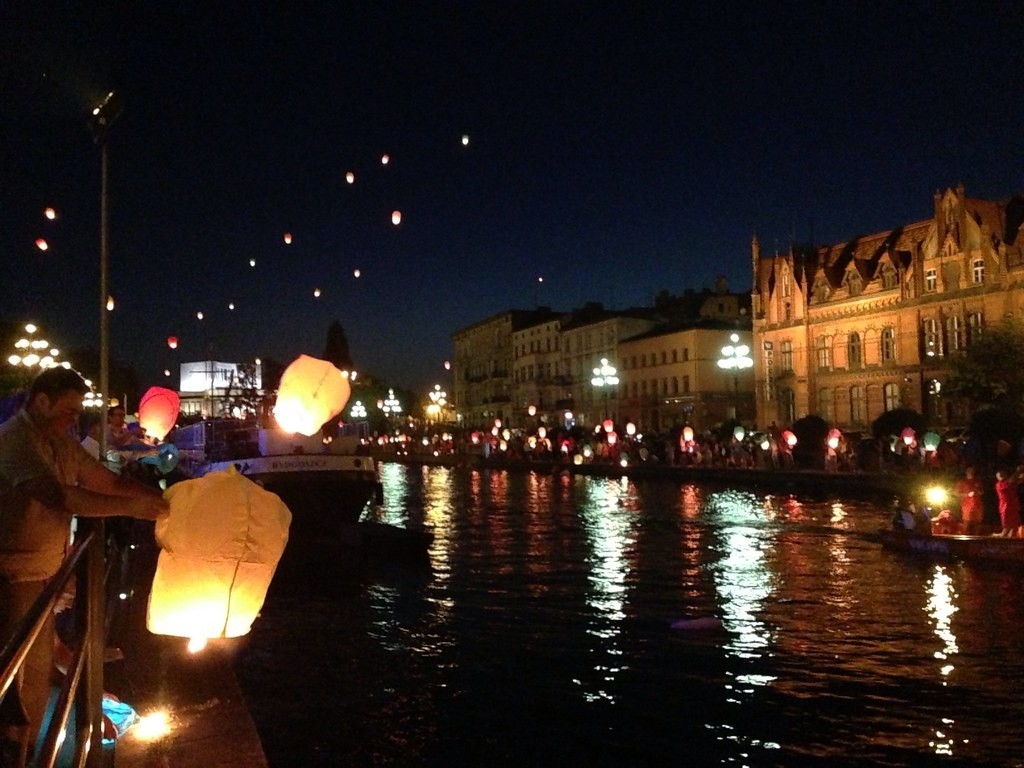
[592,358,620,419]
[716,333,753,424]
[90,90,122,468]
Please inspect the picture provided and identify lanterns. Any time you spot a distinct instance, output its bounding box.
[139,386,180,441]
[272,355,351,437]
[146,465,294,649]
[167,336,178,349]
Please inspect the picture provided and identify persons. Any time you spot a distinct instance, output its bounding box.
[360,420,961,473]
[0,366,170,768]
[893,465,1024,537]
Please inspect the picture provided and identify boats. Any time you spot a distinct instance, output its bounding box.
[878,524,1024,562]
[166,398,379,553]
